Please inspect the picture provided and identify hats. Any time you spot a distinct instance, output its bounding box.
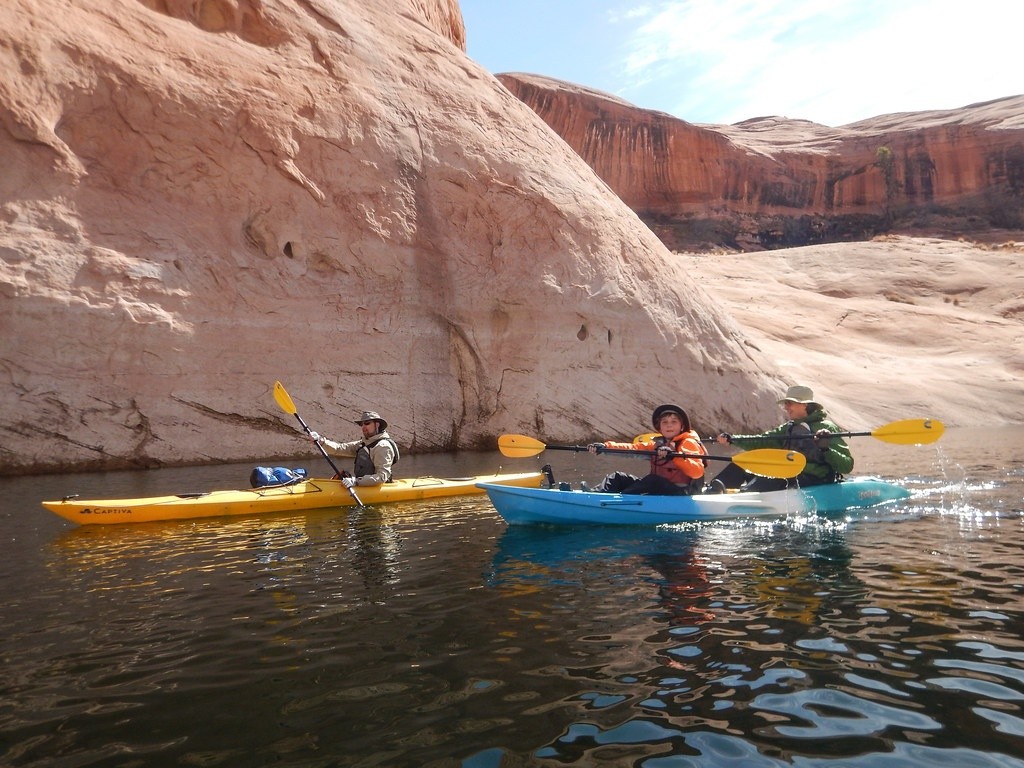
[777,386,823,411]
[354,411,388,431]
[652,404,691,434]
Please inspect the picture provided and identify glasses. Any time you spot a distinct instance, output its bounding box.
[359,421,379,426]
[783,400,794,406]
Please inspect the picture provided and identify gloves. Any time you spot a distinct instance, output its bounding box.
[341,476,357,489]
[308,431,322,445]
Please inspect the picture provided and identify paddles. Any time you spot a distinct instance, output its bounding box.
[498,432,806,479]
[273,379,365,508]
[632,417,944,446]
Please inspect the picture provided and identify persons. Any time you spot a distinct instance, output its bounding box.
[308,411,400,489]
[711,386,855,493]
[580,404,705,496]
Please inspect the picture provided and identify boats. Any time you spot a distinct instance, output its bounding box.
[475,481,914,527]
[40,472,544,527]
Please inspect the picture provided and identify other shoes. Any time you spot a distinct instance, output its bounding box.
[711,479,726,493]
[581,481,592,492]
[559,482,573,491]
